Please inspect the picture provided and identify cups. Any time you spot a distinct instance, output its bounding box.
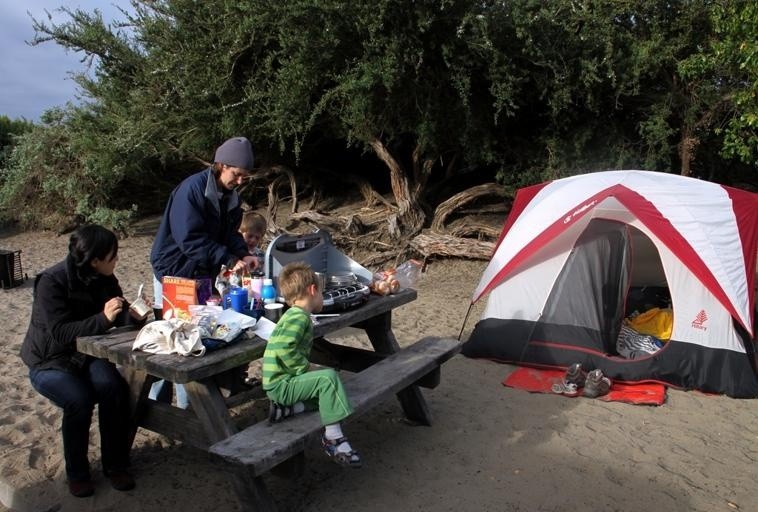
[250,271,265,301]
[221,286,248,310]
[313,272,328,294]
[263,303,284,324]
[128,300,152,321]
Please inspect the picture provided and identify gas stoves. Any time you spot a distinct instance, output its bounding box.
[276,270,370,314]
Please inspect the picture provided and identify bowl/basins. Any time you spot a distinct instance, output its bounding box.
[327,271,356,286]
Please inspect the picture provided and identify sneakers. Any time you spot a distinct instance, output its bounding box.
[102,453,136,492]
[551,363,612,398]
[64,457,95,498]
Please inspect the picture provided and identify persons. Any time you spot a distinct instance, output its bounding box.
[214,211,269,298]
[147,136,259,410]
[18,224,153,498]
[260,261,361,469]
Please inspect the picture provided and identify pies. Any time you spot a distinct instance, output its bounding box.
[375,275,400,295]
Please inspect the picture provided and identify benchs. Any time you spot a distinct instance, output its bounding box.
[84,360,169,455]
[205,332,465,511]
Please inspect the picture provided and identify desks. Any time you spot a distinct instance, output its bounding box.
[72,263,436,511]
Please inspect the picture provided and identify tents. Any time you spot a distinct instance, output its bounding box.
[455,168,757,408]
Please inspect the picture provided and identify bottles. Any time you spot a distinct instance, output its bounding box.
[260,278,276,303]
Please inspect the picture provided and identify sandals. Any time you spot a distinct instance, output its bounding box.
[267,399,294,423]
[318,430,365,470]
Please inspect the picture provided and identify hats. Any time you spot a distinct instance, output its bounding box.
[214,136,254,171]
